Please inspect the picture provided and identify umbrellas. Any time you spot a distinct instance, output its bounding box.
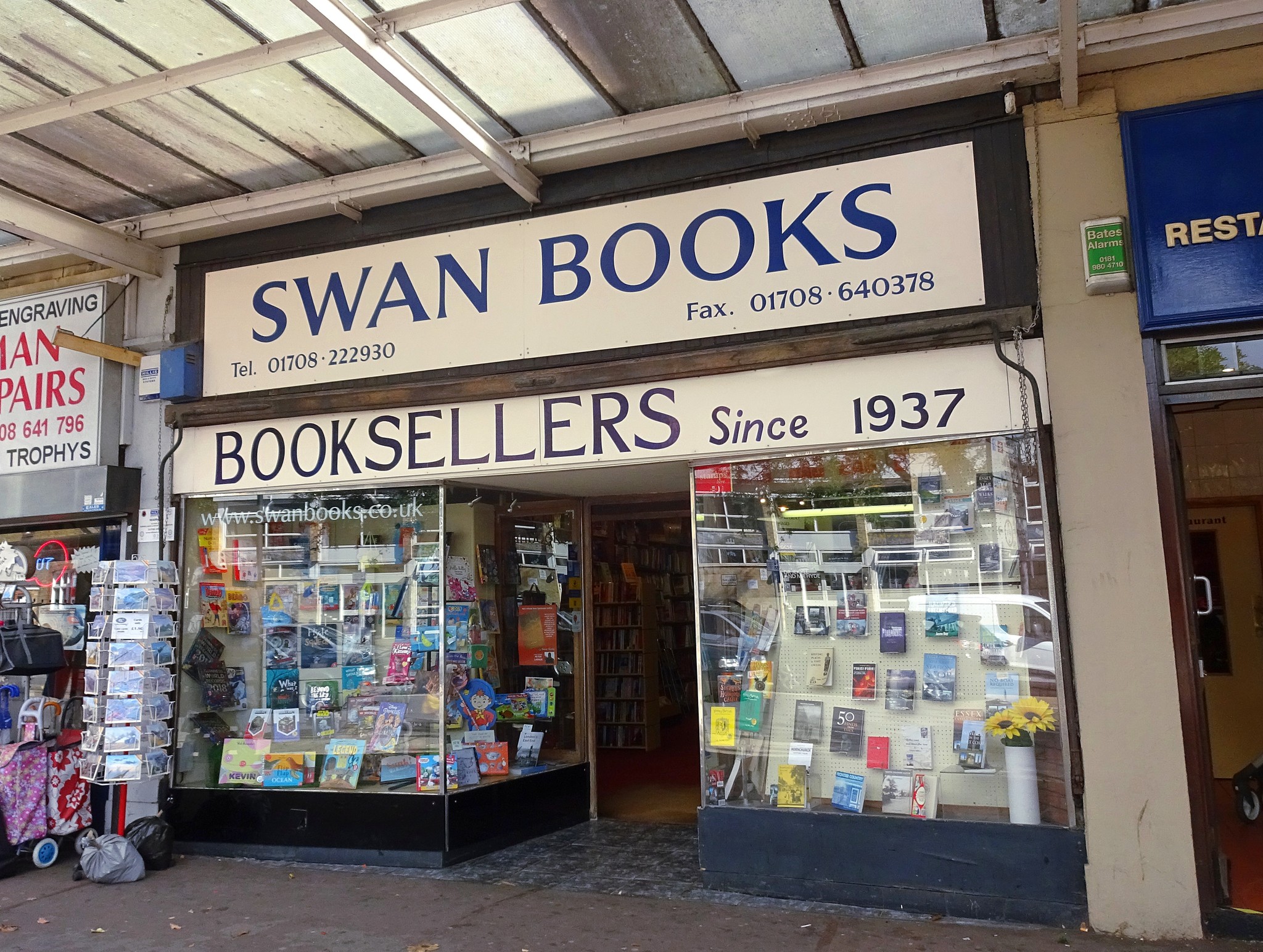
[1,683,62,745]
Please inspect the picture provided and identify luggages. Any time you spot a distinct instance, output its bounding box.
[44,696,99,856]
[0,696,60,869]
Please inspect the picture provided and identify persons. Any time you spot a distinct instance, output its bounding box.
[920,728,928,739]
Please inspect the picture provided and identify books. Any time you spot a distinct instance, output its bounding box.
[197,528,546,791]
[584,472,1022,819]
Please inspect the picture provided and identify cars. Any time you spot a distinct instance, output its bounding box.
[700,597,778,684]
[261,623,373,669]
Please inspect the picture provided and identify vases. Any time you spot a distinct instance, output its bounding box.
[1004,747,1041,825]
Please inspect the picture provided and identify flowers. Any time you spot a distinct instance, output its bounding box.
[984,699,1058,747]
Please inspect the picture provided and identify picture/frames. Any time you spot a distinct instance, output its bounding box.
[977,541,1004,574]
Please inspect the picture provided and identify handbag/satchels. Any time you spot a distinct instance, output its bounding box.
[0,608,69,677]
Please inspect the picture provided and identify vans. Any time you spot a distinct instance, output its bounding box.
[906,594,1055,679]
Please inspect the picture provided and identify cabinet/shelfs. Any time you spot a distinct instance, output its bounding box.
[658,570,695,652]
[592,520,691,573]
[594,576,661,752]
